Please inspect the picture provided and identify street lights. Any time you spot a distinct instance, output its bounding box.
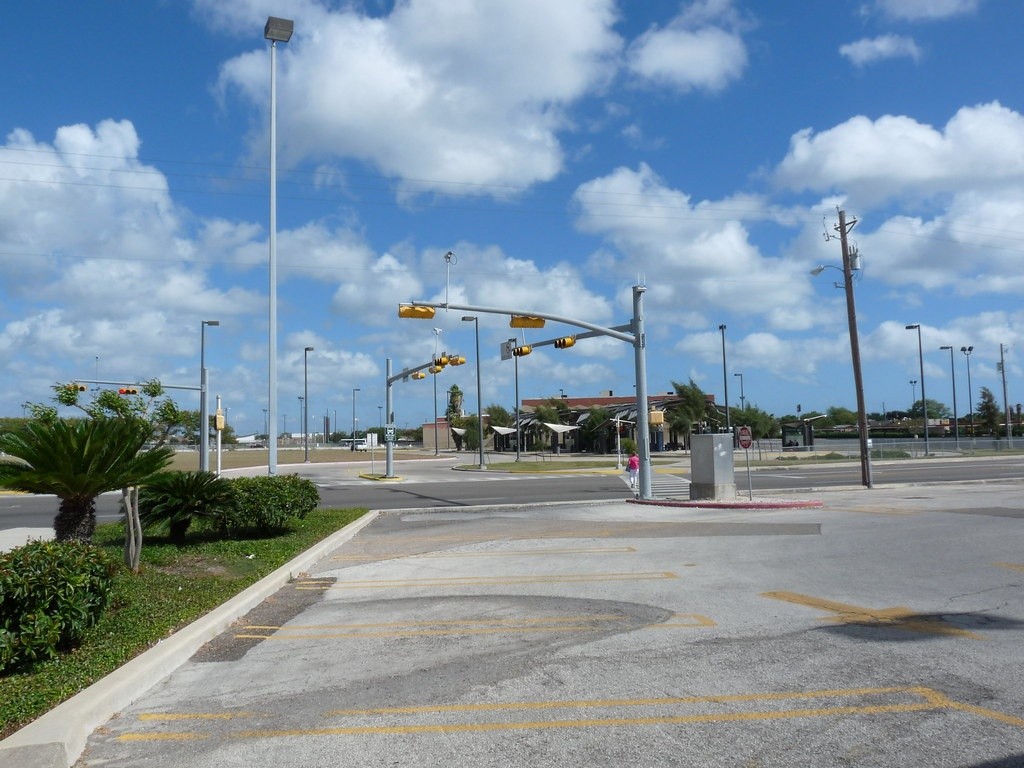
[461,316,485,466]
[297,347,315,462]
[263,16,293,477]
[507,337,521,462]
[810,264,873,489]
[734,373,744,412]
[378,406,383,427]
[199,320,219,471]
[960,346,974,435]
[910,380,917,405]
[352,389,361,451]
[906,325,929,456]
[719,324,731,432]
[263,409,267,433]
[560,389,563,402]
[940,346,959,450]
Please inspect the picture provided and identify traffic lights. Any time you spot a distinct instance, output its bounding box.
[213,414,226,431]
[68,384,88,391]
[434,357,448,367]
[554,337,576,349]
[450,356,466,366]
[428,366,443,374]
[412,372,426,380]
[120,388,138,395]
[512,346,531,357]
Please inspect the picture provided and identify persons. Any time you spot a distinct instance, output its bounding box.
[787,440,799,451]
[628,451,639,487]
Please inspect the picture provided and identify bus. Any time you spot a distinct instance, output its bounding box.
[338,439,367,452]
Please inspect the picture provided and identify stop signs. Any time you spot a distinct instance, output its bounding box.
[738,427,751,448]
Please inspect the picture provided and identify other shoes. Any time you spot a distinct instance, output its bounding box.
[634,486,636,488]
[631,484,634,488]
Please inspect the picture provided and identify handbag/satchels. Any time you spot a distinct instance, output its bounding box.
[625,465,630,472]
